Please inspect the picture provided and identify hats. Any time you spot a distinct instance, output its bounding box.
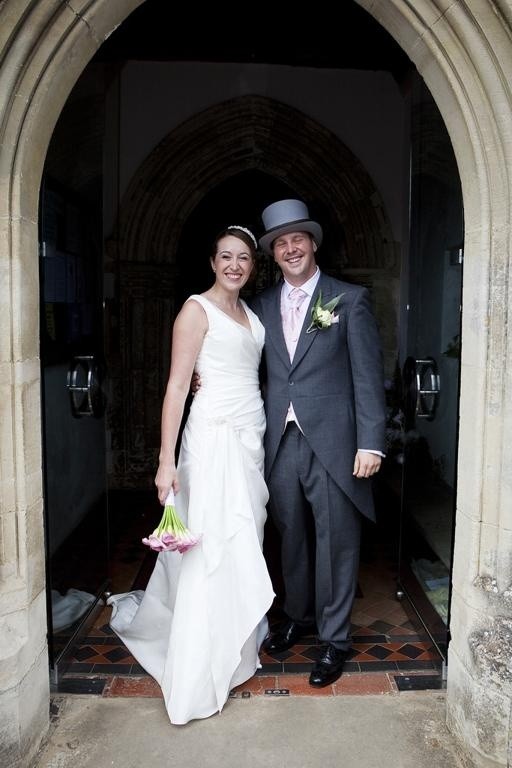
[259,199,323,256]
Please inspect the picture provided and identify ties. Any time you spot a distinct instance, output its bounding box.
[289,288,307,365]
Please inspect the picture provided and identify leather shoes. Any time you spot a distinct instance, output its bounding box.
[265,622,299,652]
[309,645,346,687]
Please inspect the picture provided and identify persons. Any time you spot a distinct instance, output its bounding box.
[105,173,275,729]
[247,196,385,695]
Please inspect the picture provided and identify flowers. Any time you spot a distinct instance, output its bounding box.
[139,470,202,560]
[296,286,350,333]
